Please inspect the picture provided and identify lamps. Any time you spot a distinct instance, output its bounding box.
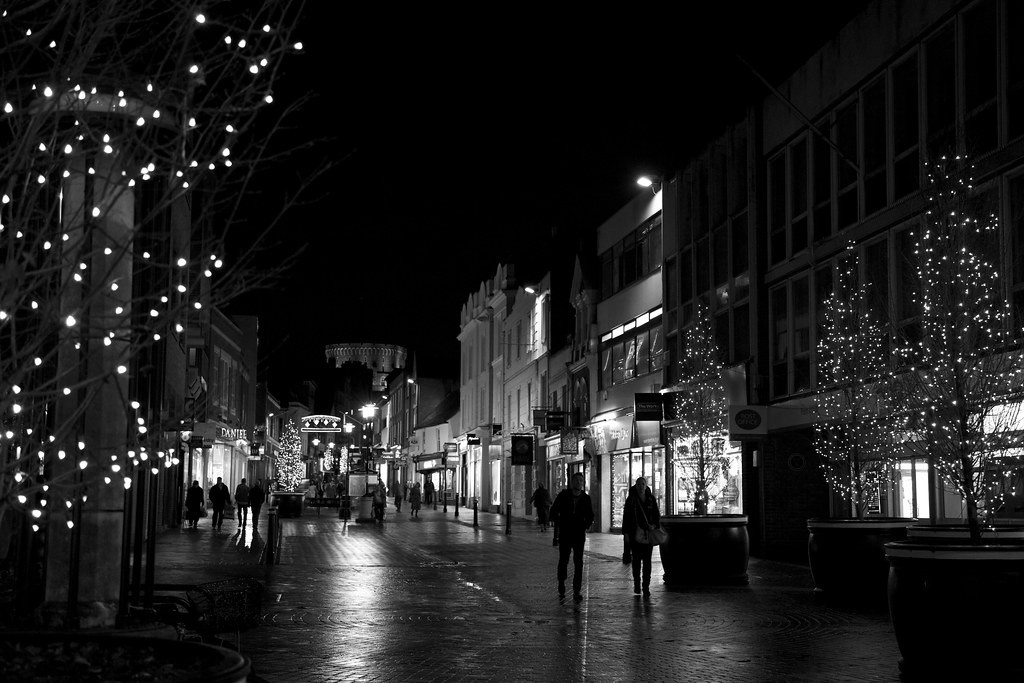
[636,174,661,194]
[524,284,540,295]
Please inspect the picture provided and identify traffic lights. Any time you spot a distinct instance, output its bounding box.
[339,423,355,519]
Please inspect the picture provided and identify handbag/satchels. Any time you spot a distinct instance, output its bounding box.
[383,509,387,520]
[373,492,382,503]
[185,509,190,521]
[200,507,208,518]
[622,533,634,565]
[635,523,670,547]
[223,504,234,519]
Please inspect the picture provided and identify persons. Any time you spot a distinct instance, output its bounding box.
[209,477,231,530]
[410,482,421,517]
[621,477,660,596]
[404,483,408,501]
[249,480,265,528]
[235,478,250,526]
[549,473,594,595]
[424,477,434,505]
[367,485,386,529]
[393,481,402,511]
[528,483,553,532]
[186,480,203,527]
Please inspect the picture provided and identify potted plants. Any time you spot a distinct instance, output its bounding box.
[0,0,335,683]
[268,418,306,520]
[658,152,1024,683]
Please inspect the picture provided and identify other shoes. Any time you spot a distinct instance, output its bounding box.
[634,582,641,594]
[642,589,650,595]
[572,591,584,602]
[557,580,565,595]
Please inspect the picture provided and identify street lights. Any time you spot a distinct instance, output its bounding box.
[312,439,321,516]
[355,404,380,524]
[328,442,335,508]
